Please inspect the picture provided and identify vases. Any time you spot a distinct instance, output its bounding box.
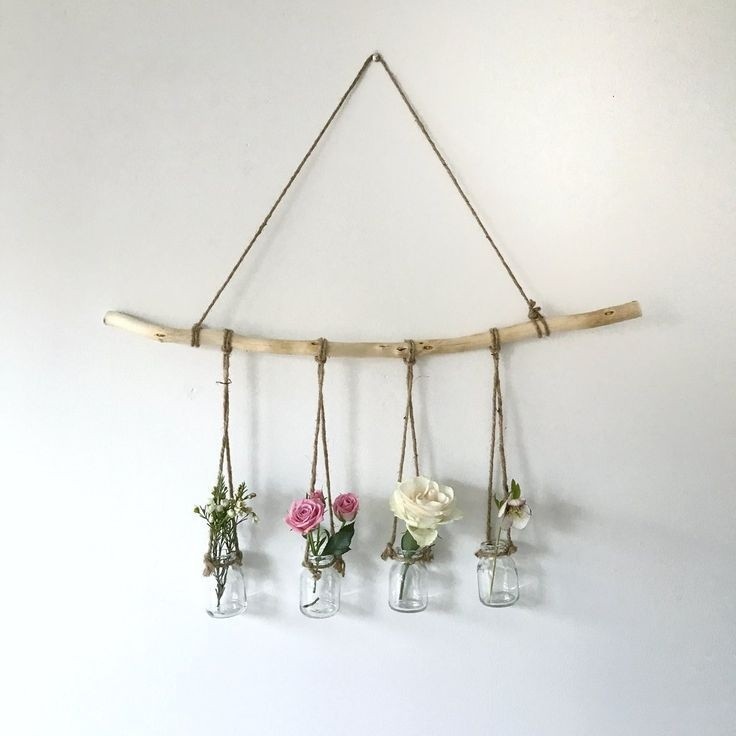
[388,546,429,612]
[477,541,519,607]
[203,548,247,617]
[298,547,341,619]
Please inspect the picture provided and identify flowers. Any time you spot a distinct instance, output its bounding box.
[287,488,359,558]
[490,479,531,545]
[390,477,461,559]
[197,475,259,548]
